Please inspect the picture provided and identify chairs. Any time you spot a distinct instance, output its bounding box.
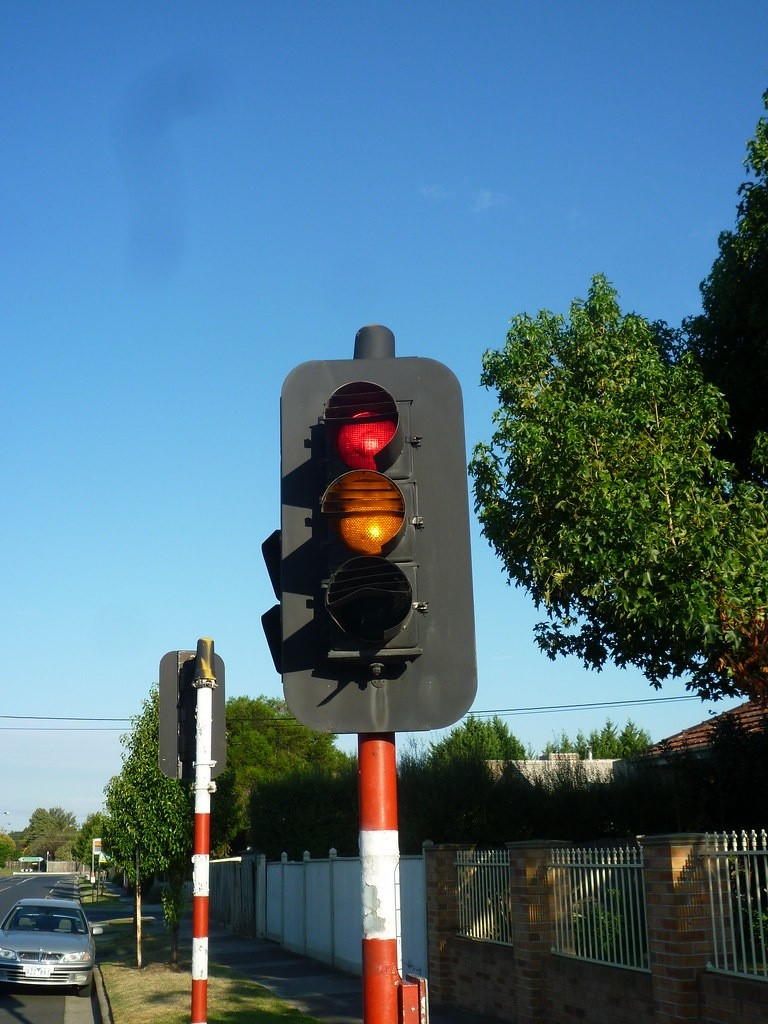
[18,916,33,930]
[53,919,72,933]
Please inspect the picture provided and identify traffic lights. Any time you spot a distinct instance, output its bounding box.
[280,356,478,734]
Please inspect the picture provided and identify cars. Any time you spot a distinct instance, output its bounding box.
[0,897,105,997]
